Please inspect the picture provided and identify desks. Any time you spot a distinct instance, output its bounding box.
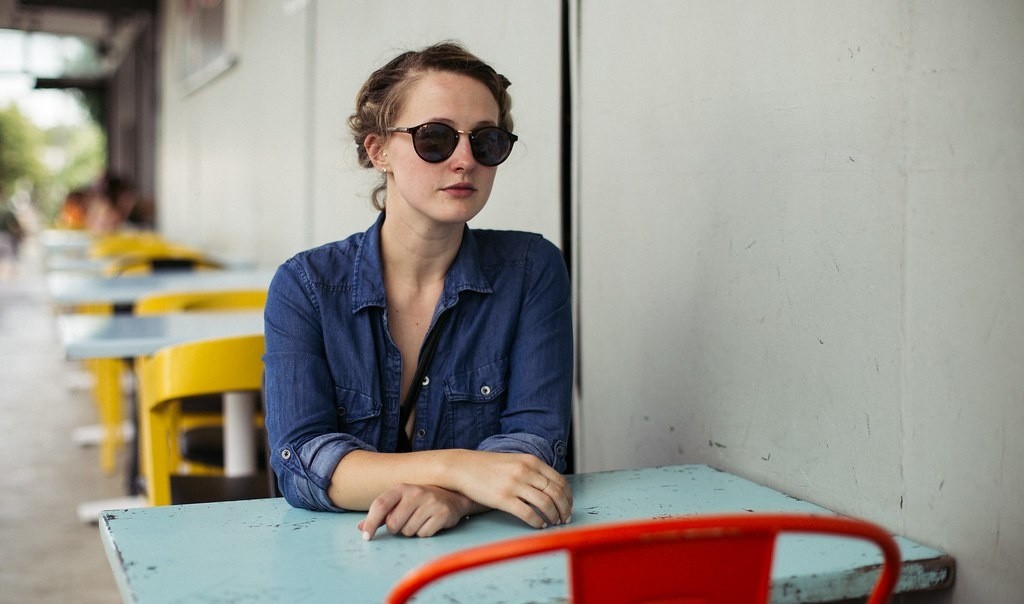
[64,311,269,523]
[100,462,956,604]
[37,227,278,446]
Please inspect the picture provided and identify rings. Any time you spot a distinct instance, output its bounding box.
[541,478,549,491]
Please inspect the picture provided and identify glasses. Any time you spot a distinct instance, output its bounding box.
[387,122,518,166]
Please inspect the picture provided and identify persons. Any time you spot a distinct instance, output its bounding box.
[264,43,574,542]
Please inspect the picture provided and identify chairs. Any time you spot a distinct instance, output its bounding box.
[135,334,274,506]
[387,513,902,604]
[54,218,267,471]
